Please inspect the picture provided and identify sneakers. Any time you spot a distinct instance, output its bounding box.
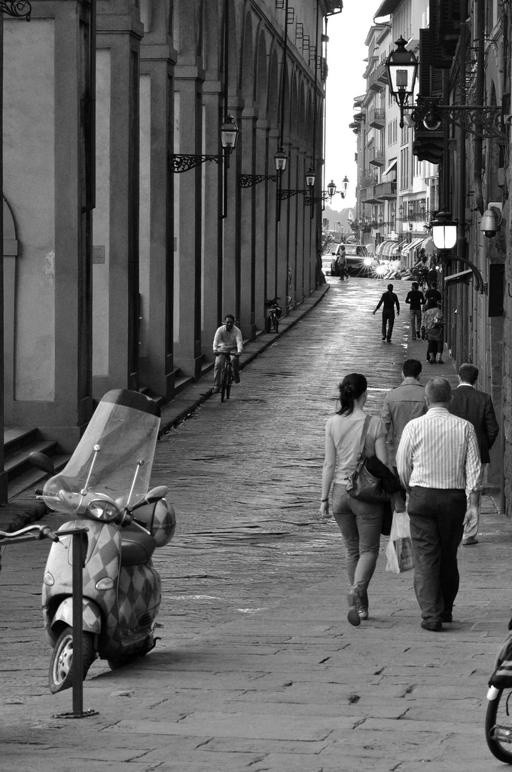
[234,376,240,384]
[430,360,436,364]
[346,590,361,626]
[212,387,220,393]
[358,606,367,619]
[461,536,478,546]
[438,359,445,364]
[387,339,391,343]
[382,336,385,340]
[422,618,443,632]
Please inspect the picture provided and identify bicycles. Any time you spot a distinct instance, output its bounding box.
[264,296,282,335]
[214,350,240,404]
[483,620,510,764]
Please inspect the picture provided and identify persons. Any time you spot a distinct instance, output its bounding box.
[319,371,389,627]
[337,245,348,281]
[372,284,400,342]
[405,246,447,365]
[379,359,430,513]
[447,364,499,546]
[395,377,484,633]
[212,314,243,394]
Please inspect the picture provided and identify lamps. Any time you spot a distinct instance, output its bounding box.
[382,31,512,141]
[274,164,318,203]
[428,205,487,296]
[168,111,243,175]
[322,175,350,200]
[313,178,339,207]
[236,140,291,188]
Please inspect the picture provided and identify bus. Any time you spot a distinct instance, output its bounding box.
[321,254,336,263]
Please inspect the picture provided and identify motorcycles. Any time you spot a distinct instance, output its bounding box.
[35,386,178,695]
[380,260,415,282]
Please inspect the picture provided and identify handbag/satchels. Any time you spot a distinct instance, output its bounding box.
[345,416,398,505]
[384,509,415,572]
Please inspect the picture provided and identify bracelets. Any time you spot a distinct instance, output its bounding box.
[320,498,328,501]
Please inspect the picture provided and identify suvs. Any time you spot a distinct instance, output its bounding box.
[330,244,374,279]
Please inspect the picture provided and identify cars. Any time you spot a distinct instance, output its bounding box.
[346,235,357,244]
[321,262,335,276]
[367,251,385,278]
